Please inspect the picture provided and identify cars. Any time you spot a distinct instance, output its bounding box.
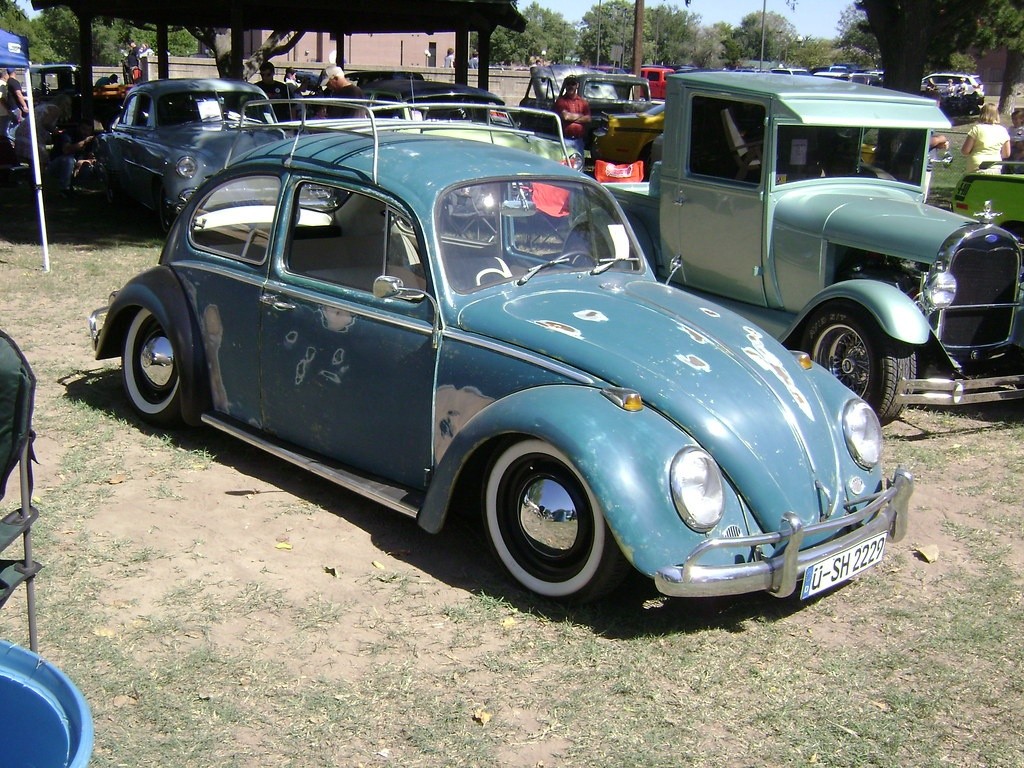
[520,65,665,142]
[90,99,914,606]
[563,72,1024,427]
[771,66,884,87]
[591,104,666,184]
[641,67,675,99]
[591,66,626,74]
[952,160,1024,244]
[344,71,423,88]
[72,78,340,233]
[362,79,584,220]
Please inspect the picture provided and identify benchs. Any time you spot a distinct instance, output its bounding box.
[209,231,420,274]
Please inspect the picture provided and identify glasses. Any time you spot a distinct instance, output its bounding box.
[565,81,577,86]
[260,72,273,76]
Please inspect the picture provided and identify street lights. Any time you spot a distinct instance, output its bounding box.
[541,50,546,66]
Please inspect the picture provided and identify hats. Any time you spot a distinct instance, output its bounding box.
[7,68,15,74]
[564,75,580,83]
[111,74,118,83]
[320,66,343,86]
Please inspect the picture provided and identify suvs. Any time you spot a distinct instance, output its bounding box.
[921,71,984,106]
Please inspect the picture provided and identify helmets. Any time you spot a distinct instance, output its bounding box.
[948,79,953,83]
[960,77,966,82]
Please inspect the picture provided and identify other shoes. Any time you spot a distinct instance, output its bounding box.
[59,186,74,200]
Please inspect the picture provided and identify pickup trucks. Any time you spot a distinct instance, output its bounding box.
[30,65,135,130]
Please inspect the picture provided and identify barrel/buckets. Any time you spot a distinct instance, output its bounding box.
[862,143,876,164]
[0,640,94,768]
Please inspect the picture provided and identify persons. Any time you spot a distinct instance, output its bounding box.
[95,74,120,90]
[960,103,1024,176]
[253,61,365,119]
[444,48,479,69]
[958,77,968,96]
[531,60,542,67]
[926,77,940,89]
[948,78,955,97]
[0,68,29,125]
[556,76,592,160]
[923,90,948,206]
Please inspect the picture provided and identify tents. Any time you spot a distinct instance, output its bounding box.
[0,29,51,271]
